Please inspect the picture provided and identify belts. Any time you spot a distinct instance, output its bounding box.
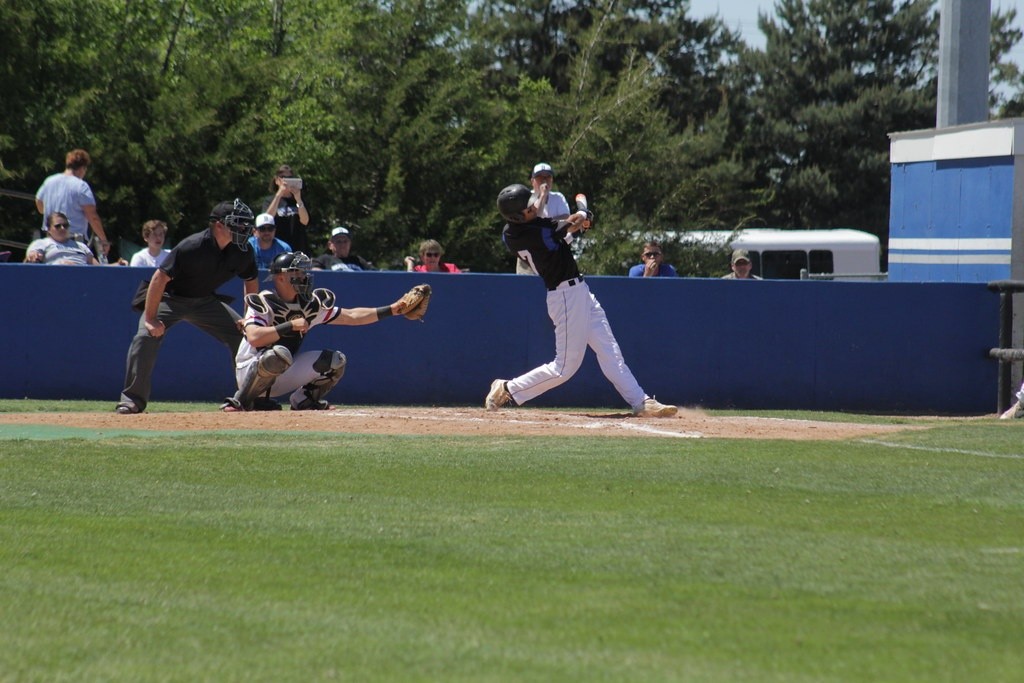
[549,274,585,292]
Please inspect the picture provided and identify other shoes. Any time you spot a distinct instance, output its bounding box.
[117,406,129,414]
[255,397,283,409]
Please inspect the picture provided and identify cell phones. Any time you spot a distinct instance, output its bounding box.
[283,179,302,189]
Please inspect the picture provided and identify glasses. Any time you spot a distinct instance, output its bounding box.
[643,250,662,258]
[49,223,69,229]
[259,226,275,232]
[422,252,441,259]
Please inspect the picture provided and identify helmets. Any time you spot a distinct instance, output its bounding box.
[497,184,538,222]
[270,252,297,274]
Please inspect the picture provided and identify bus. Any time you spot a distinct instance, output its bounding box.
[570,227,881,279]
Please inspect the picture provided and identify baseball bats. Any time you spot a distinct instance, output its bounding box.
[575,193,591,229]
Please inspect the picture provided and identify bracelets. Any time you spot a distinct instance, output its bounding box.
[296,202,304,208]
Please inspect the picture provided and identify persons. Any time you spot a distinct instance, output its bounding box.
[247,213,292,269]
[486,182,679,421]
[310,227,387,273]
[220,249,432,411]
[719,249,763,280]
[26,211,129,267]
[129,220,173,268]
[515,163,570,274]
[260,164,309,251]
[628,240,677,278]
[404,239,461,273]
[114,198,284,414]
[35,149,110,260]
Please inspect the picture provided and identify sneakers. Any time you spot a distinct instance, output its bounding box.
[220,402,240,412]
[633,397,677,417]
[290,399,328,409]
[485,379,513,410]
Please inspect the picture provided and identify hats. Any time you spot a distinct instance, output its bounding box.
[532,163,554,177]
[256,213,276,227]
[210,201,252,234]
[732,249,752,266]
[332,227,349,237]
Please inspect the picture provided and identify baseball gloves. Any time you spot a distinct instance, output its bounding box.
[397,284,431,321]
